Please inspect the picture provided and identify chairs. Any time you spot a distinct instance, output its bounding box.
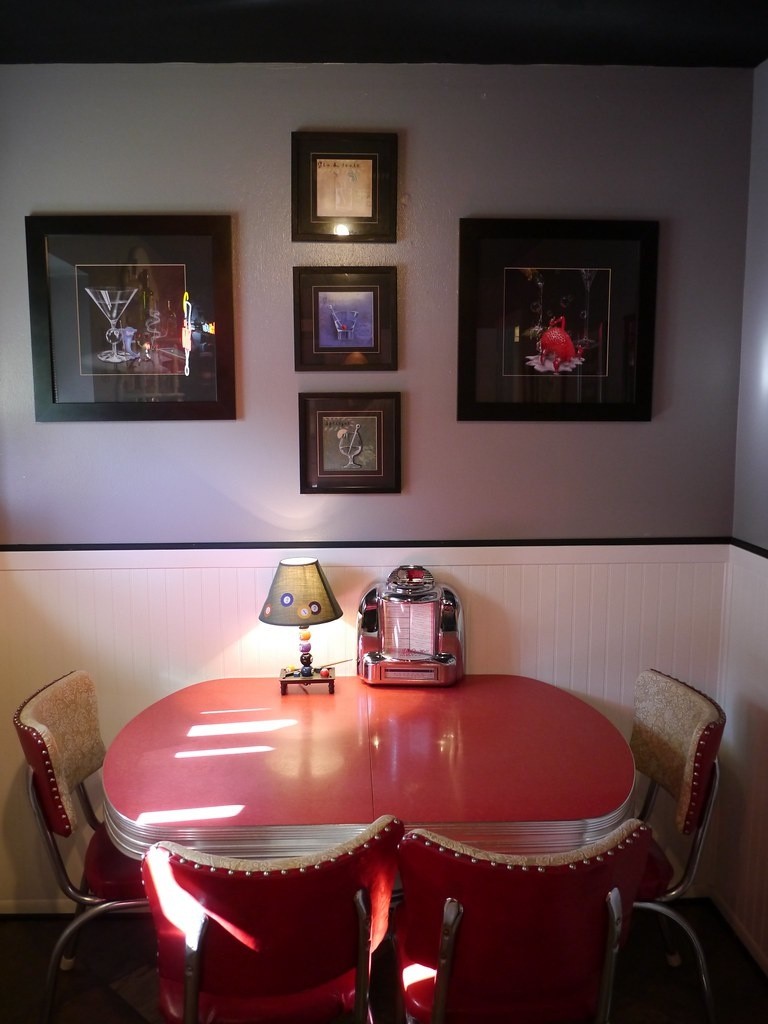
[633,668,726,1024]
[397,818,652,1024]
[139,814,405,1024]
[13,668,150,1024]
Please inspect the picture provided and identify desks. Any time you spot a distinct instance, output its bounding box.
[367,674,636,855]
[102,676,373,862]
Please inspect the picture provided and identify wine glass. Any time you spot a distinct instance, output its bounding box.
[85,287,138,362]
[339,432,362,469]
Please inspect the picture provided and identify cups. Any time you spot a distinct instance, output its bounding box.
[333,310,359,340]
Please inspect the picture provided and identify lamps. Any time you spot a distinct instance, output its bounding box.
[258,556,343,695]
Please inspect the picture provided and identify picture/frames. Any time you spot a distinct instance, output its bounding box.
[456,218,659,422]
[25,215,237,422]
[291,131,398,244]
[292,266,399,372]
[298,392,402,494]
